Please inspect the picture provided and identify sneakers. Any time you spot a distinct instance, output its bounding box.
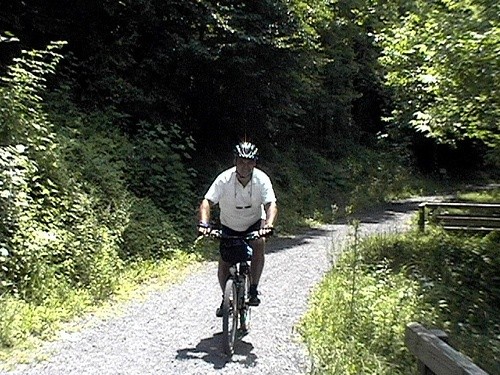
[215,295,234,317]
[248,284,261,304]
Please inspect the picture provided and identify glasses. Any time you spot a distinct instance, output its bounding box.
[235,192,252,209]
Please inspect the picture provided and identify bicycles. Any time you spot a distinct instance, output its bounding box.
[197,227,273,355]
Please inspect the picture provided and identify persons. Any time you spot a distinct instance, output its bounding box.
[198,141,278,317]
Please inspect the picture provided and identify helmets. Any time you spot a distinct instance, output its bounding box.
[232,141,260,161]
